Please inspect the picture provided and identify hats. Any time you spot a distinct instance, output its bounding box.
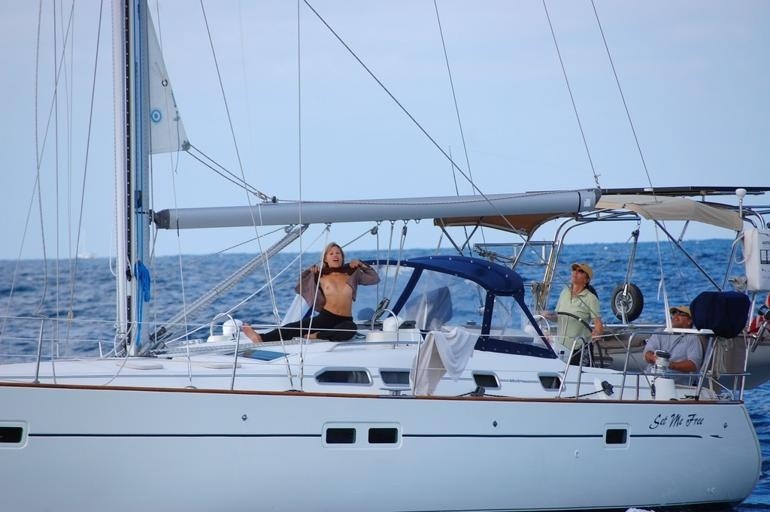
[571,263,593,281]
[669,305,691,317]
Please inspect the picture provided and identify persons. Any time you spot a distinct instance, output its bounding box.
[641,304,705,386]
[529,260,602,365]
[240,241,380,347]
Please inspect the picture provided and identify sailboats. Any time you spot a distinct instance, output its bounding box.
[0,0,770,512]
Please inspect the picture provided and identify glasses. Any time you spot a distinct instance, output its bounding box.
[672,311,689,317]
[573,268,586,274]
